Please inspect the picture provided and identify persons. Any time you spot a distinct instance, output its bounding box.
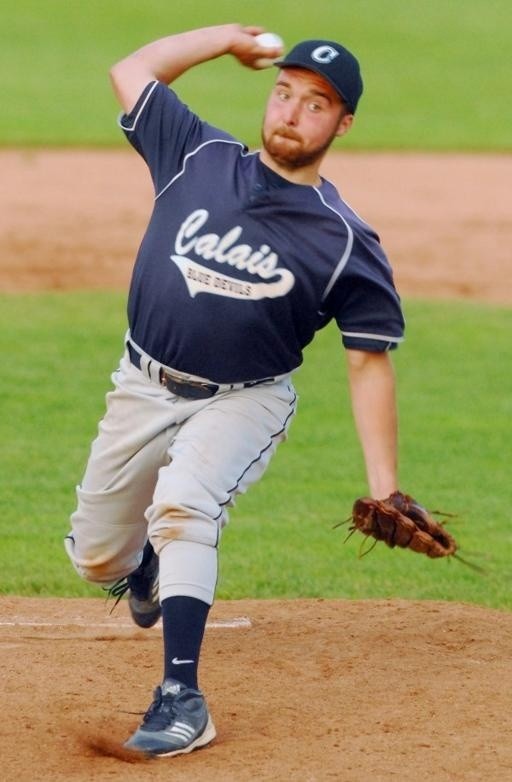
[61,24,458,763]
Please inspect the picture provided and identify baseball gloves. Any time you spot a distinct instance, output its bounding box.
[352,491,456,557]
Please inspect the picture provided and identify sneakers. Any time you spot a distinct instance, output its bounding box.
[121,676,216,758]
[108,555,162,628]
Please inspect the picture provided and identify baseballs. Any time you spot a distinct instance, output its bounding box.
[255,34,281,58]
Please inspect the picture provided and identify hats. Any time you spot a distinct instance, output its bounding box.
[273,39,362,116]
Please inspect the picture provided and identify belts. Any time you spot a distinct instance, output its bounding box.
[126,340,274,400]
